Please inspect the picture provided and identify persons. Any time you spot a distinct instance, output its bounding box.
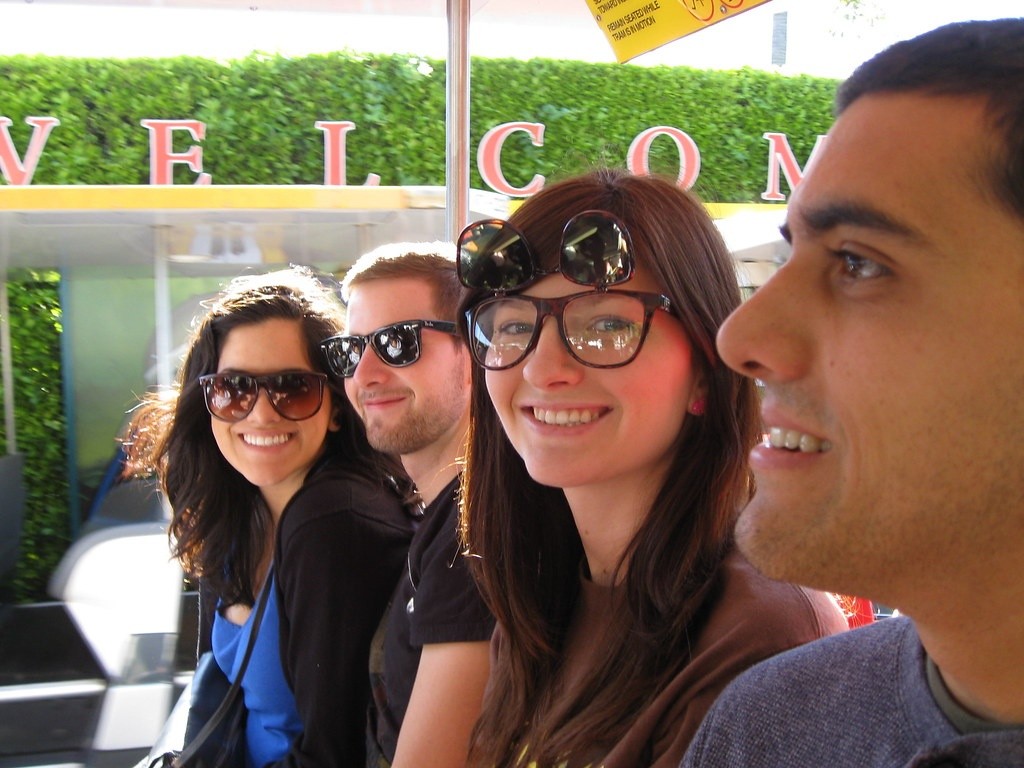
[679,14,1023,764]
[147,266,412,767]
[463,168,848,767]
[330,242,504,768]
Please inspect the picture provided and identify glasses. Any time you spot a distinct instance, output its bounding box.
[457,210,682,370]
[198,371,336,421]
[321,319,462,377]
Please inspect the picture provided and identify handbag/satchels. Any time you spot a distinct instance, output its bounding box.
[147,651,245,768]
